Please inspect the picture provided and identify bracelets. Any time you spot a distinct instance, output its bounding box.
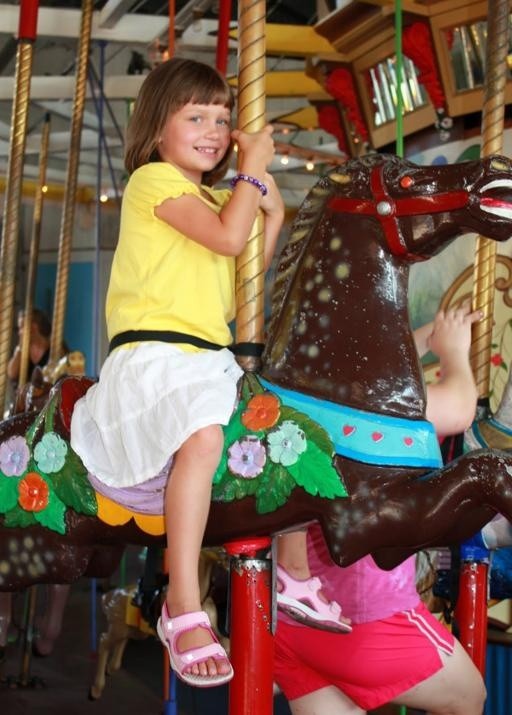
[231,174,267,196]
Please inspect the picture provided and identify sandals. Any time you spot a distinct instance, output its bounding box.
[155,597,236,689]
[275,563,353,634]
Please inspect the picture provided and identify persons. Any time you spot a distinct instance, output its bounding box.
[71,56,352,687]
[0,584,70,664]
[274,302,487,715]
[7,307,72,416]
